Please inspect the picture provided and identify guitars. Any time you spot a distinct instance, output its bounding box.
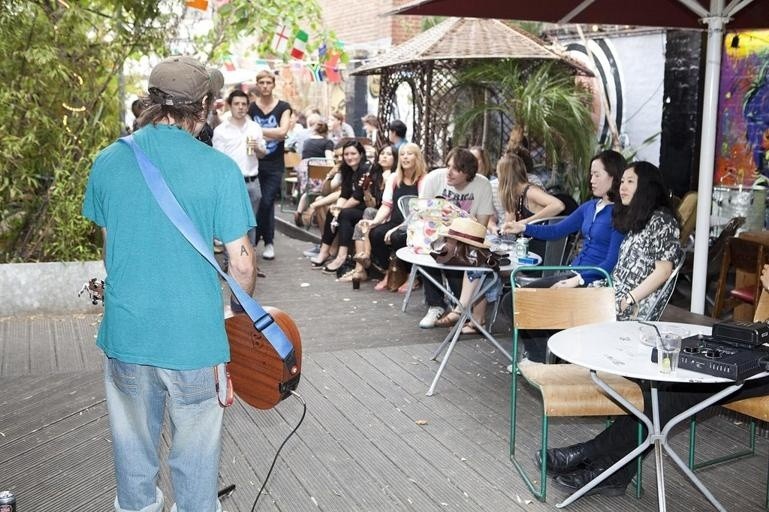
[77,278,302,409]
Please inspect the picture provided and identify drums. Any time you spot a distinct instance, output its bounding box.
[710,184,769,239]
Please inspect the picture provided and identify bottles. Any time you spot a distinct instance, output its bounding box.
[750,186,767,231]
[246,136,254,156]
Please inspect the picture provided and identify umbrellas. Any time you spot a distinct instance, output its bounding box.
[378,1,769,315]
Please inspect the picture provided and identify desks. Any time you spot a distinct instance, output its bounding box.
[549,318,768,512]
[395,246,542,401]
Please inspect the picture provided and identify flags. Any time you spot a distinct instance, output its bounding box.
[184,1,344,86]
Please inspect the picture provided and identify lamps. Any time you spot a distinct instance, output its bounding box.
[731,34,742,49]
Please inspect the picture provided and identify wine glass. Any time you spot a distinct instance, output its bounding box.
[712,185,752,226]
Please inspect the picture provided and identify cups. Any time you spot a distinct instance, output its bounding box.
[353,273,360,289]
[655,332,682,374]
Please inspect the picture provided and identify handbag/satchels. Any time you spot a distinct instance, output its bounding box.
[430,238,492,265]
[407,198,477,254]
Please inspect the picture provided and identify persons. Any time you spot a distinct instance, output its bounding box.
[294,114,427,292]
[195,72,293,259]
[80,56,257,512]
[413,126,684,375]
[284,109,343,198]
[535,263,769,496]
[130,98,148,131]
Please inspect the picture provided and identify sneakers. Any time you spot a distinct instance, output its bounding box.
[304,244,370,282]
[294,211,305,228]
[263,244,276,261]
[421,305,445,329]
[507,356,532,374]
[399,279,421,293]
[374,279,389,290]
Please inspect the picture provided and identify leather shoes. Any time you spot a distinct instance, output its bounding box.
[550,456,631,496]
[533,442,591,475]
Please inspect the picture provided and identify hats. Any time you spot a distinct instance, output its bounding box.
[148,56,225,105]
[438,218,492,248]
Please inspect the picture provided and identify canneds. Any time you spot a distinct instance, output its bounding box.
[248,136,254,156]
[1,490,16,512]
[515,237,528,258]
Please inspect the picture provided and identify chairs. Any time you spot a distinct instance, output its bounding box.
[675,193,698,245]
[680,217,748,305]
[508,265,642,499]
[520,214,584,276]
[645,247,685,321]
[397,195,417,220]
[711,232,768,319]
[685,284,769,472]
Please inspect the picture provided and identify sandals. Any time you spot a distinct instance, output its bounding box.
[435,311,487,335]
